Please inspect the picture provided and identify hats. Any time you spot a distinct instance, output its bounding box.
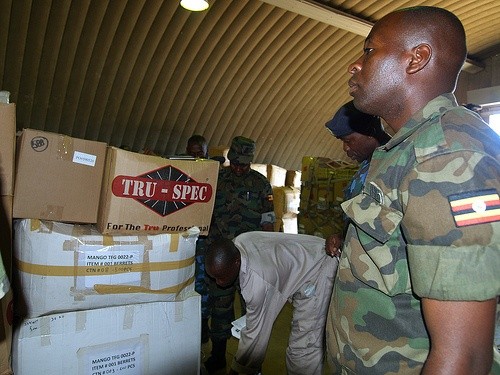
[325,99,393,145]
[227,136,256,164]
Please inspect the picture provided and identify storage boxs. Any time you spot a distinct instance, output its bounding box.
[0,90,301,375]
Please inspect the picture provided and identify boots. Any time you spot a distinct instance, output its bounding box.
[204,338,227,369]
[201,318,210,344]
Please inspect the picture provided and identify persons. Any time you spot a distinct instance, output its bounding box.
[201,136,277,368]
[325,99,392,258]
[186,134,225,166]
[324,6,500,375]
[204,231,338,375]
